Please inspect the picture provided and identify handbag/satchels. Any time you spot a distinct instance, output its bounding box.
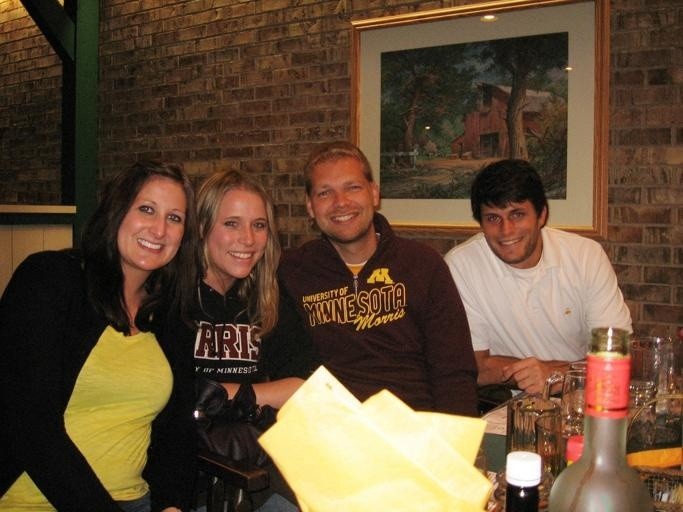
[193,381,279,512]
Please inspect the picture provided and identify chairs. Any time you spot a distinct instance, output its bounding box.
[174,445,269,512]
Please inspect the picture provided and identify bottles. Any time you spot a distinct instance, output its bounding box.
[546,326,655,512]
[504,450,542,512]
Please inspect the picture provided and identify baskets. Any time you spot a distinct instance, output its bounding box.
[627,394,683,512]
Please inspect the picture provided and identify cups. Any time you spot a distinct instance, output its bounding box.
[628,337,674,415]
[505,360,587,507]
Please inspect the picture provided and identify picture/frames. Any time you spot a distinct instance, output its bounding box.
[350,0,610,240]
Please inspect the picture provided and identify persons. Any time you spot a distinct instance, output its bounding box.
[0,157,200,510]
[177,166,320,512]
[274,139,481,422]
[440,154,633,398]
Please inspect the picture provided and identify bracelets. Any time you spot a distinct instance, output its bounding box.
[568,363,579,388]
